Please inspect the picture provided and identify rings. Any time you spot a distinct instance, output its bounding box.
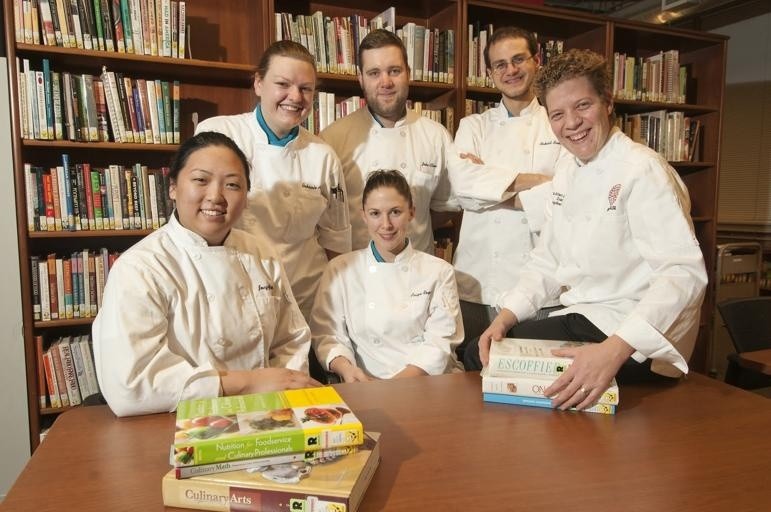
[579,388,589,397]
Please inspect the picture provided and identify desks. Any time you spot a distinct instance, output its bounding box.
[1,367,770,511]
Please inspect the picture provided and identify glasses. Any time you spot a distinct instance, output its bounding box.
[491,54,534,70]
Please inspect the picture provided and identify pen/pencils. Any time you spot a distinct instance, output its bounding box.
[330,187,345,205]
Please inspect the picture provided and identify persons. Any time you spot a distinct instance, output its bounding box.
[190,43,358,348]
[450,31,577,368]
[100,134,311,418]
[320,28,452,260]
[308,167,471,388]
[478,49,700,414]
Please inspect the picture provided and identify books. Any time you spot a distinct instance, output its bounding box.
[159,386,383,511]
[613,43,703,164]
[34,333,98,408]
[13,0,185,59]
[482,337,619,417]
[24,154,179,231]
[15,57,180,144]
[273,5,454,138]
[467,20,567,116]
[32,248,119,322]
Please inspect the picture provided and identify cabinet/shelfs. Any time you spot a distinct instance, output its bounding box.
[713,241,762,378]
[2,0,271,457]
[272,3,461,320]
[611,18,729,373]
[460,0,609,122]
